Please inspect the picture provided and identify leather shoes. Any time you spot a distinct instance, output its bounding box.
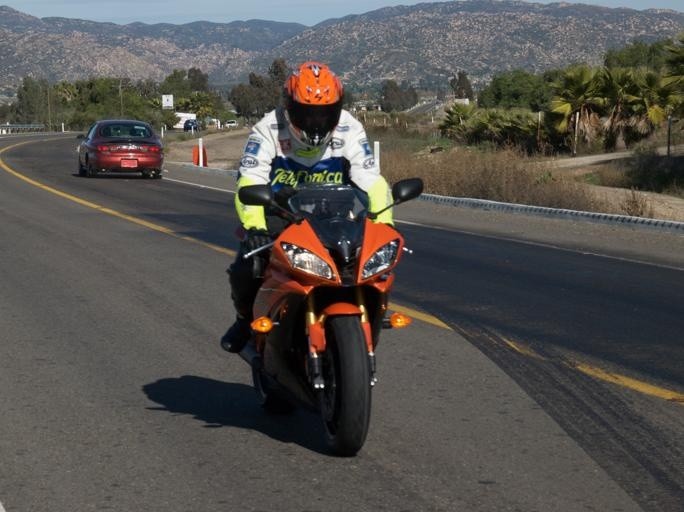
[221,321,252,353]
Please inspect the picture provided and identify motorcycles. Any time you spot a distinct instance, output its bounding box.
[237,173,426,457]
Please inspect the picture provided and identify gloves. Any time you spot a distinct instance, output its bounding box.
[241,226,274,253]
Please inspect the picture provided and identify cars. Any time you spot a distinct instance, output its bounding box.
[75,119,164,179]
[183,118,200,132]
[225,119,239,129]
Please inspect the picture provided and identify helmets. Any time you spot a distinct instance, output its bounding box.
[282,60,344,149]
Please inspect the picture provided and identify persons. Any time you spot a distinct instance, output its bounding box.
[220,62,396,354]
